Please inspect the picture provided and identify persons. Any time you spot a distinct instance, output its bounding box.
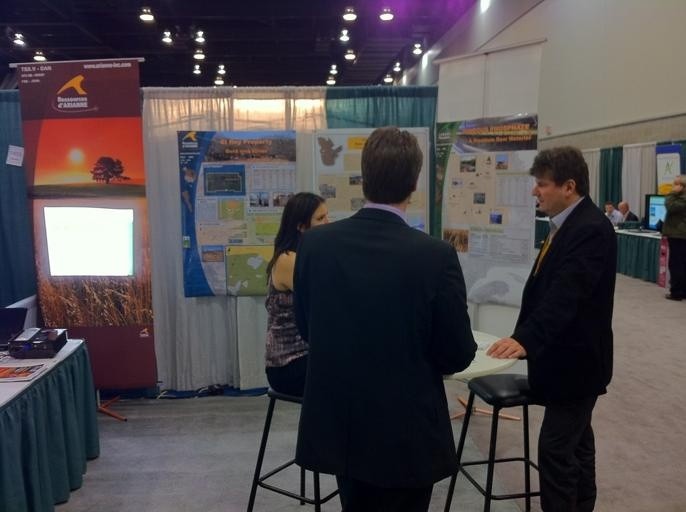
[484,145,620,512]
[603,199,624,226]
[662,175,686,301]
[292,127,479,511]
[262,191,333,397]
[617,199,640,223]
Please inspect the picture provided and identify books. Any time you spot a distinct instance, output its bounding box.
[0,363,49,382]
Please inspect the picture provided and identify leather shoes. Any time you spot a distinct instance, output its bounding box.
[666,293,681,301]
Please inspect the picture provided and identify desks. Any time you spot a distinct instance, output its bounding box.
[535,216,671,287]
[441,329,522,421]
[0,335,101,510]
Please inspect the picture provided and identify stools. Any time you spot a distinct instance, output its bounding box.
[442,372,544,512]
[248,386,341,511]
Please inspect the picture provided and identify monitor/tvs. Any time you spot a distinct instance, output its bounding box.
[645,195,669,230]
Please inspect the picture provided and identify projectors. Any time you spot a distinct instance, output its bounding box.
[8,328,68,359]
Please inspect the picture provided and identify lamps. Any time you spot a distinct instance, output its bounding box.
[0,25,49,63]
[325,0,424,87]
[138,0,228,87]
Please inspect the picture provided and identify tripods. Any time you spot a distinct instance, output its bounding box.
[450,394,520,423]
[94,388,128,423]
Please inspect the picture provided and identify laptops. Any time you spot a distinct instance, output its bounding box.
[0,308,27,350]
